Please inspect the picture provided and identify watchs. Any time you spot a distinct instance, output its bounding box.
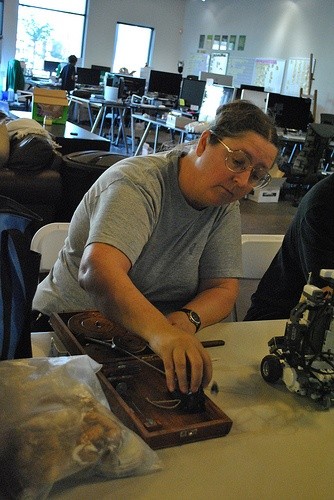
[176,308,201,333]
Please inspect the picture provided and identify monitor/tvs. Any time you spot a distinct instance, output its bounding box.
[148,70,236,125]
[76,65,111,86]
[266,94,311,135]
[44,61,60,77]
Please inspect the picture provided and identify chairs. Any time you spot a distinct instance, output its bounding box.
[28,223,285,323]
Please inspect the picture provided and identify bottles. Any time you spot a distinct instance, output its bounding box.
[43,115,52,126]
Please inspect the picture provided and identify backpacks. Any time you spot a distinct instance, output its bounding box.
[0,193,44,362]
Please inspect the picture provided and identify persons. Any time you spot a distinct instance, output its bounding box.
[243,172,334,321]
[15,100,279,393]
[59,55,77,121]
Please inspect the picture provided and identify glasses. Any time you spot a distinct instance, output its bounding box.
[209,128,272,191]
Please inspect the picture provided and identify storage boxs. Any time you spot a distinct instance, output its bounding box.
[32,87,69,124]
[166,114,194,129]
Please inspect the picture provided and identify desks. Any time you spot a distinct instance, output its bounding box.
[65,94,334,173]
[28,317,334,500]
[8,110,111,155]
